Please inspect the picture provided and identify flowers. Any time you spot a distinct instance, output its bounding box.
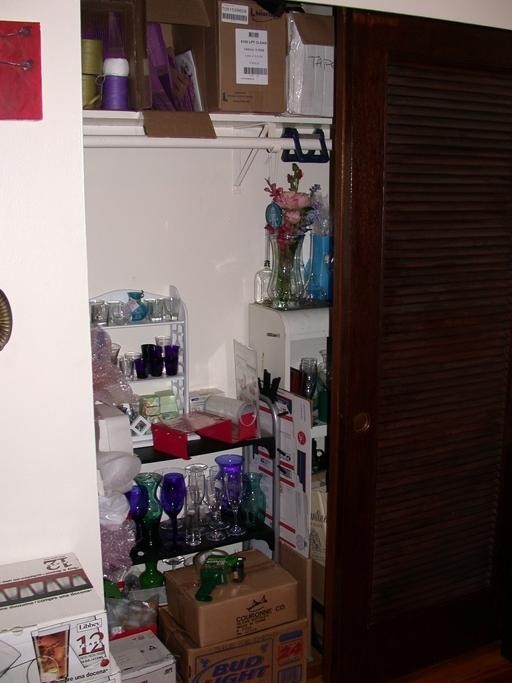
[262,163,322,301]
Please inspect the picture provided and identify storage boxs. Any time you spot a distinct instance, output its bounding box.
[156,607,312,682]
[2,552,112,683]
[81,0,337,139]
[163,549,299,648]
[311,420,328,456]
[88,653,122,682]
[107,630,176,681]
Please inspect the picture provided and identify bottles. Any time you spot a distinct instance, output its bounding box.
[300,273,327,305]
[231,558,244,583]
[126,290,146,322]
[139,559,164,589]
[253,260,272,305]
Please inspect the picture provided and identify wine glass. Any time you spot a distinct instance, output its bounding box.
[128,455,266,558]
[298,350,328,422]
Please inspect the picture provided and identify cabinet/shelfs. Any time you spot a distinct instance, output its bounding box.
[248,302,331,488]
[89,286,190,449]
[97,390,282,568]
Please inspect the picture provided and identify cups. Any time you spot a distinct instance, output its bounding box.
[112,335,180,381]
[147,297,181,323]
[91,300,127,326]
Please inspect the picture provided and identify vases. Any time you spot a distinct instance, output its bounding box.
[268,232,308,311]
